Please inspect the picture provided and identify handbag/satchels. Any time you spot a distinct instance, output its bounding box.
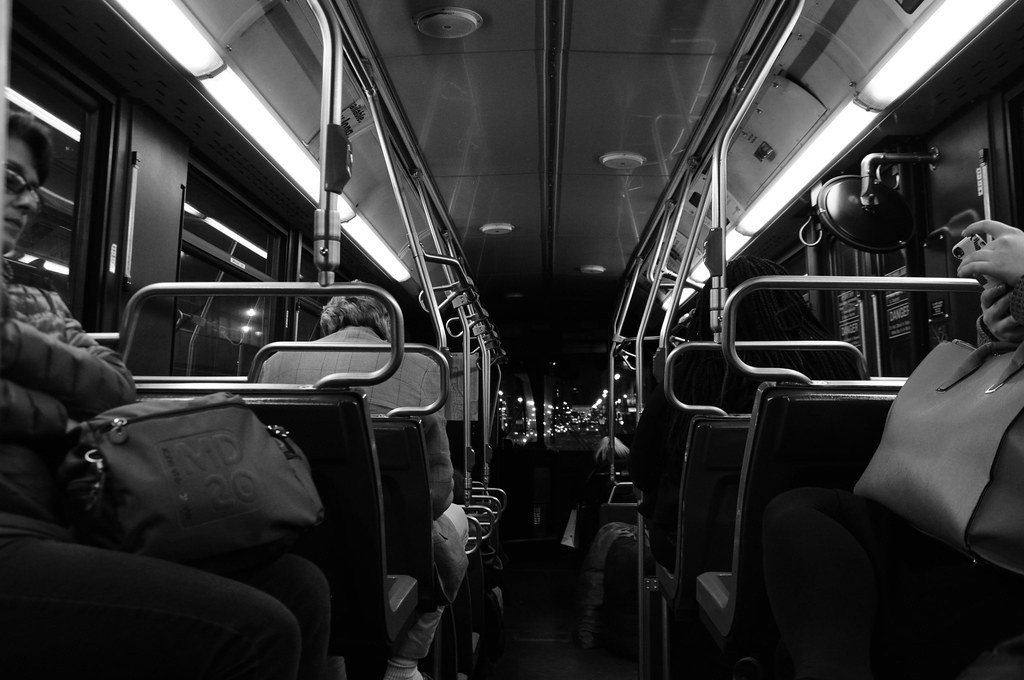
[854,338,1024,577]
[44,391,323,559]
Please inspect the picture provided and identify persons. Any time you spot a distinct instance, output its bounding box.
[259,220,1024,680]
[0,112,331,679]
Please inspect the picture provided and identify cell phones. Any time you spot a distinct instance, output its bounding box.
[952,233,1005,289]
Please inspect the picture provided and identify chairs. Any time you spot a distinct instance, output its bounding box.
[116,282,507,680]
[606,273,986,680]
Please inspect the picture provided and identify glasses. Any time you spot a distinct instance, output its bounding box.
[6,166,42,214]
